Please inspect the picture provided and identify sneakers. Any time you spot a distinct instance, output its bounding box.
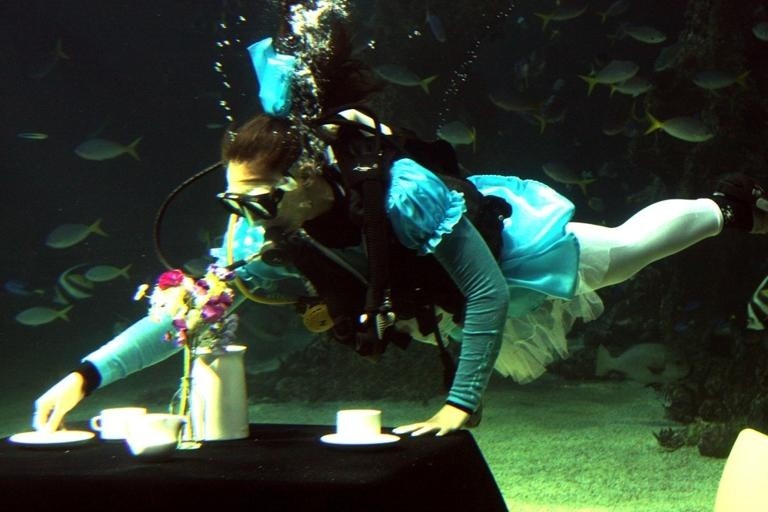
[700,173,753,232]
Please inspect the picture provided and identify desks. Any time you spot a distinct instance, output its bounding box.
[0,424,509,512]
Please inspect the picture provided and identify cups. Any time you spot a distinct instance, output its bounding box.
[335,409,383,441]
[90,406,148,441]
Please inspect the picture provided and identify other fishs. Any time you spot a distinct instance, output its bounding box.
[592,343,691,388]
[746,275,768,332]
[5,127,145,327]
[23,36,72,80]
[346,0,768,213]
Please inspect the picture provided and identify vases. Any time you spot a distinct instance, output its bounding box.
[185,346,250,442]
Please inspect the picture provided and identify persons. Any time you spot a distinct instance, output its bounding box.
[31,1,767,437]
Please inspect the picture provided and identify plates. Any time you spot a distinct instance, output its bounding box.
[319,432,402,447]
[8,429,96,446]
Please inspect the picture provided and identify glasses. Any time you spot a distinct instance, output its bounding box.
[216,148,309,220]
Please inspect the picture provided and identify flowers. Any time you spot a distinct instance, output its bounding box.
[134,264,239,355]
